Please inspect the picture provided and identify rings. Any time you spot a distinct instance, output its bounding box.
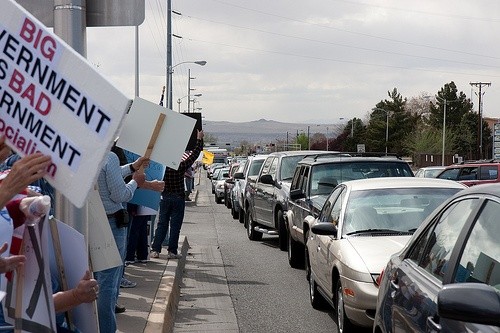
[92,287,96,292]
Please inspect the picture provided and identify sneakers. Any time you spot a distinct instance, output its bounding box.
[167,252,183,259]
[125,260,135,264]
[150,251,159,258]
[120,276,136,287]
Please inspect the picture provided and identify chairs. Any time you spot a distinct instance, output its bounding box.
[352,207,378,231]
[318,178,338,194]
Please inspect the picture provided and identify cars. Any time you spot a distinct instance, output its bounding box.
[371,183,500,333]
[415,166,450,179]
[202,148,268,223]
[305,177,475,333]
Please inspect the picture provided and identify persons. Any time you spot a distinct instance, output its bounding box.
[0,130,204,333]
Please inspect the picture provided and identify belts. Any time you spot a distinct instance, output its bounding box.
[107,214,115,219]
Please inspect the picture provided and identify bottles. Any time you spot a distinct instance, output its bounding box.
[23,194,51,227]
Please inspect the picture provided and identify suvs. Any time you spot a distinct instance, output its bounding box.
[281,152,430,271]
[246,150,343,242]
[433,160,500,188]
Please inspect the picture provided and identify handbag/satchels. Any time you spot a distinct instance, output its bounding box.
[115,209,130,227]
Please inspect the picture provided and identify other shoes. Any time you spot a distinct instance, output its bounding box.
[115,303,126,313]
[185,196,191,201]
[135,260,152,263]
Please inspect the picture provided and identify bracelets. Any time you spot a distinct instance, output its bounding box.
[130,164,135,173]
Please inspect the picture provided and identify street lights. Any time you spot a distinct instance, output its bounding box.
[371,107,406,157]
[423,95,462,165]
[168,60,207,110]
[177,93,203,114]
[287,117,356,151]
